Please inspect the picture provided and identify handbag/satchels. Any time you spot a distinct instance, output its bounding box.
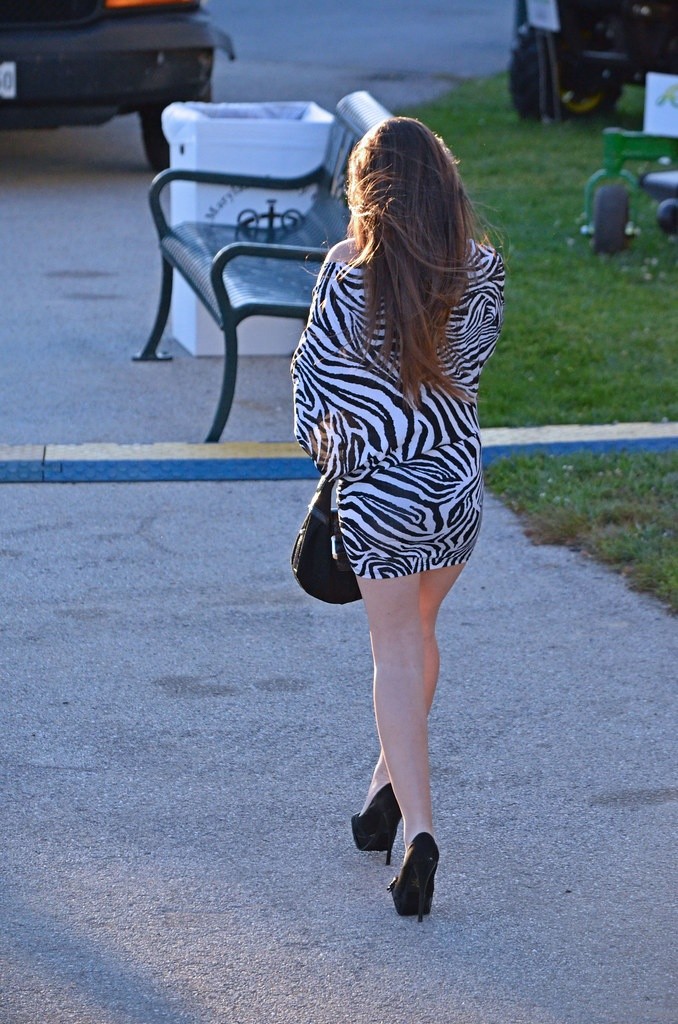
[293,469,366,604]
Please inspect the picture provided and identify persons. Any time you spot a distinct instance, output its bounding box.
[291,119,508,925]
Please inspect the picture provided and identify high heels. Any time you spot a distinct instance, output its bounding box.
[351,783,402,866]
[388,832,440,922]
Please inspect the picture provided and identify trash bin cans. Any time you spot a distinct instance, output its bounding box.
[160,100,341,360]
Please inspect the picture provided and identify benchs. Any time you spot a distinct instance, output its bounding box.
[130,88,398,446]
